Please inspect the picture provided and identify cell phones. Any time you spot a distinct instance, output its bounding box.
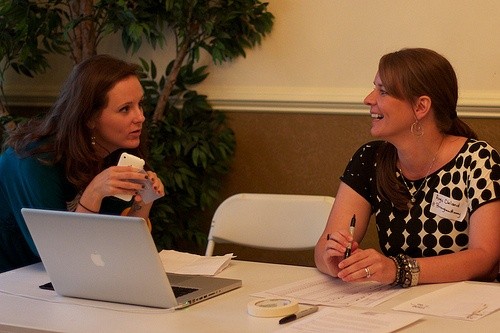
[113,152,145,202]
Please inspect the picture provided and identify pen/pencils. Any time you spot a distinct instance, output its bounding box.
[345,214,357,258]
[278,306,319,325]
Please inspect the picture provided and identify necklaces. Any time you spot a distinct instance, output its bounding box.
[398,136,444,202]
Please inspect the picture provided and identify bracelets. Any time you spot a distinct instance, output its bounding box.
[389,254,420,288]
[78,201,99,214]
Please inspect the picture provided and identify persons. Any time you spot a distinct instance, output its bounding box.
[0,54,165,273]
[315,48,500,282]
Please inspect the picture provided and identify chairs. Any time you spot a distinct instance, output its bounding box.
[205,193,335,260]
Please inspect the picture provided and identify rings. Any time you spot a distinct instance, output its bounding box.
[364,267,371,279]
[327,233,331,240]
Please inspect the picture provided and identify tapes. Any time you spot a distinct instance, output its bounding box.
[247,296,299,318]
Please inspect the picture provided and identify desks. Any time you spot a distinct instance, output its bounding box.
[0,251,500,333]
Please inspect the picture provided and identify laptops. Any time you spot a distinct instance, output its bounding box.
[20,207,243,310]
